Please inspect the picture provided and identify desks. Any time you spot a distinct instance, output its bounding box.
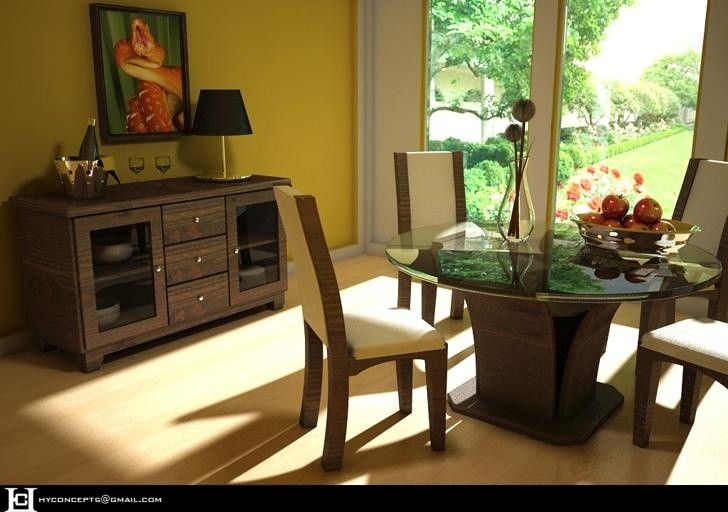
[386,221,723,447]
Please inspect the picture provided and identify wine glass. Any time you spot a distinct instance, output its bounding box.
[128,157,145,190]
[155,155,171,189]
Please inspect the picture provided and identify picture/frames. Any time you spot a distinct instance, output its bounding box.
[89,3,192,144]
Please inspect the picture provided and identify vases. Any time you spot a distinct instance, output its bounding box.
[496,237,533,290]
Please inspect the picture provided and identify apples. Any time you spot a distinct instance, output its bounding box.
[626,269,650,283]
[594,268,620,279]
[584,195,676,231]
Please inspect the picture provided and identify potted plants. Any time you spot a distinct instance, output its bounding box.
[497,99,537,243]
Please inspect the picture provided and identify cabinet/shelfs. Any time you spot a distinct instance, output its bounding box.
[10,176,291,372]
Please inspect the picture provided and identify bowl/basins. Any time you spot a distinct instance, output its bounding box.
[571,214,702,257]
[96,297,121,329]
[94,244,136,264]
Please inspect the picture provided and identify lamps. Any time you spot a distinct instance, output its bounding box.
[191,88,252,181]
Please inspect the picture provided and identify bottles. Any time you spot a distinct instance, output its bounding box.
[79,117,99,160]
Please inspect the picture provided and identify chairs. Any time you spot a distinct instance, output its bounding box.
[394,152,467,326]
[272,186,448,473]
[634,157,728,447]
